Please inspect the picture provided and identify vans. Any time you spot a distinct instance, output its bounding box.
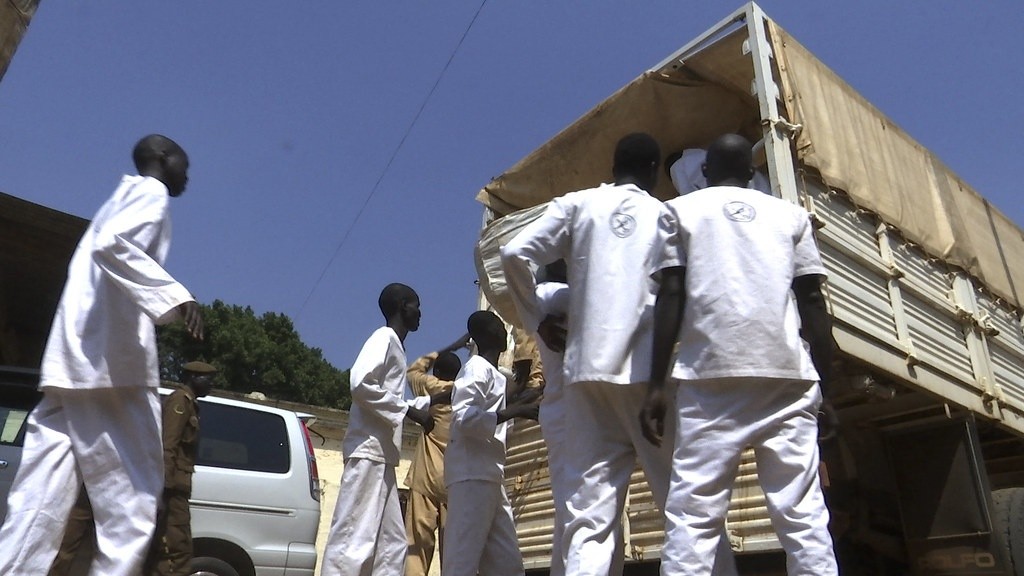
[0,361,327,576]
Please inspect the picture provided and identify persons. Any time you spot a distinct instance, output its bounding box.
[320,283,435,576]
[538,258,570,576]
[501,132,738,576]
[442,310,539,576]
[143,361,217,576]
[664,148,772,196]
[0,134,204,576]
[48,486,98,576]
[404,350,470,576]
[639,133,841,576]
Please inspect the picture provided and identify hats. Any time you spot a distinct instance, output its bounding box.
[183,361,220,380]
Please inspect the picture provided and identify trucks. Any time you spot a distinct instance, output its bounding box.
[447,1,1024,576]
